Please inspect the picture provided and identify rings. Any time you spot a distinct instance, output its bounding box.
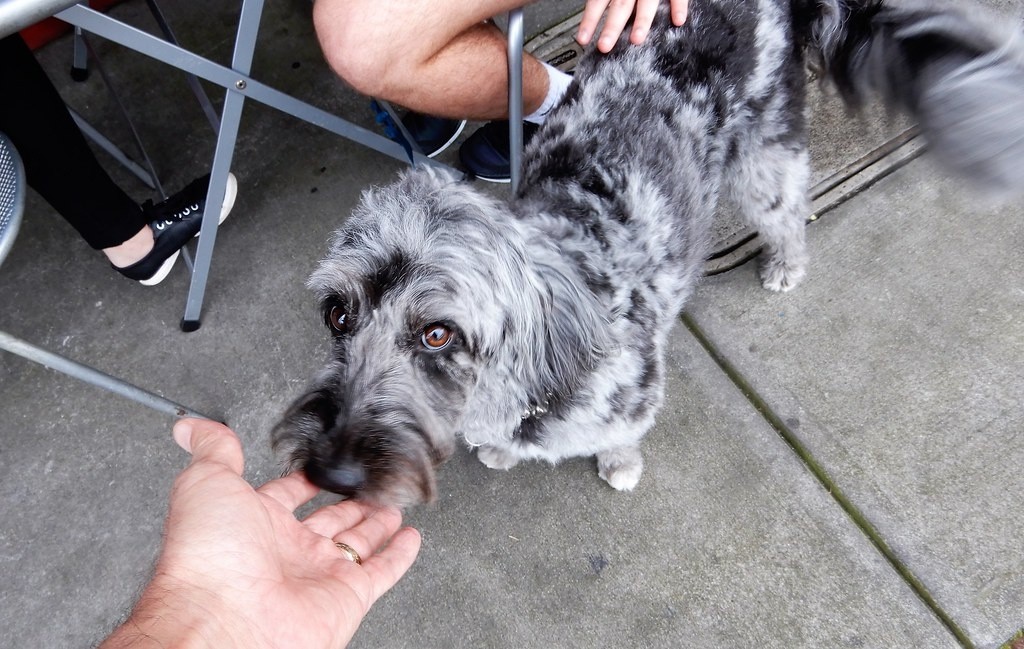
[335,542,361,564]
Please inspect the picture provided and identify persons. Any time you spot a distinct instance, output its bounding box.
[93,417,422,649]
[311,1,689,183]
[0,32,238,285]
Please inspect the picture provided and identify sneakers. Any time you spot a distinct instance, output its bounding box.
[392,110,468,159]
[459,70,576,183]
[110,172,238,286]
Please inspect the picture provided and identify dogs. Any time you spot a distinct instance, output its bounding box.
[269,1,1024,508]
